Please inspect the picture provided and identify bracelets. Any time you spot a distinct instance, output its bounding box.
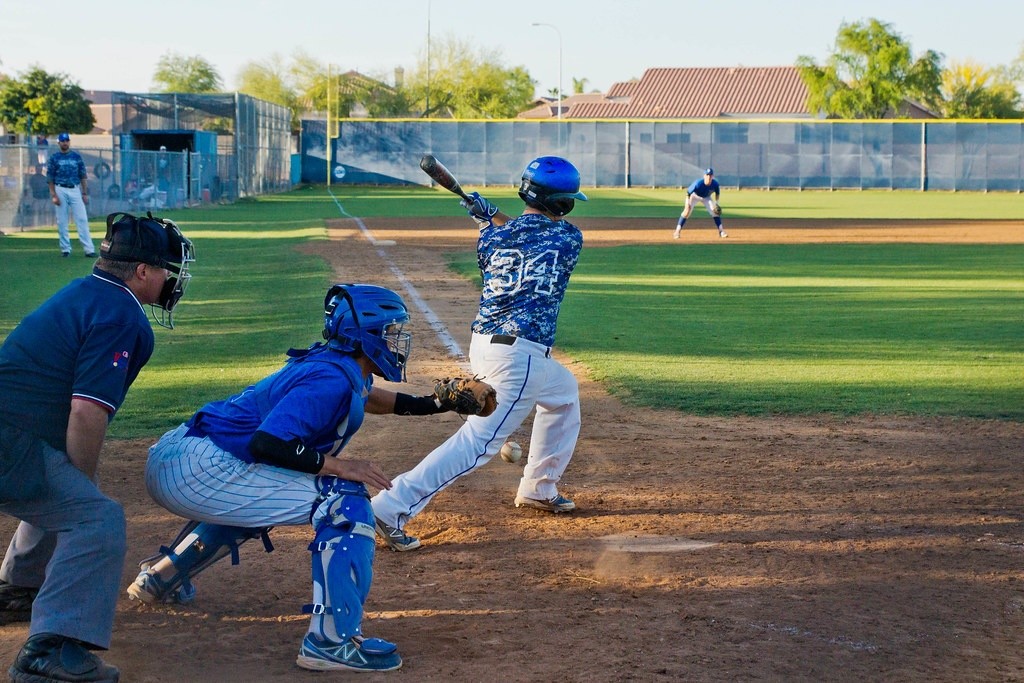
[82,193,87,195]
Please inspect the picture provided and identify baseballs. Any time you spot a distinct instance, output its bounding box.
[501,442,522,464]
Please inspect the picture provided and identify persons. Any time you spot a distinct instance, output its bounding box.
[18,164,52,226]
[37,132,48,166]
[47,133,100,257]
[8,127,16,144]
[673,169,728,240]
[0,211,193,683]
[372,156,588,552]
[151,146,171,191]
[128,284,496,671]
[1,167,17,188]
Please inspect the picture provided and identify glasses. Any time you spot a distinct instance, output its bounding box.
[60,140,68,142]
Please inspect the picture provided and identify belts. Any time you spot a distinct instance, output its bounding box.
[490,334,550,358]
[55,183,74,188]
[183,427,206,437]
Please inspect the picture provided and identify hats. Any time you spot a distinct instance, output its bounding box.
[704,168,713,175]
[58,133,69,141]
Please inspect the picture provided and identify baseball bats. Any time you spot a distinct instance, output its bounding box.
[419,154,472,203]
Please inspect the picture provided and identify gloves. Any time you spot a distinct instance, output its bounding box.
[460,193,498,236]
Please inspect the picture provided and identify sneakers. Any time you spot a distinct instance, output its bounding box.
[514,494,576,512]
[372,513,421,551]
[6,634,120,683]
[296,630,403,672]
[127,565,184,606]
[1,576,40,625]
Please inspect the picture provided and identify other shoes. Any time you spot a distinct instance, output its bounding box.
[719,231,728,238]
[62,252,98,258]
[673,232,680,240]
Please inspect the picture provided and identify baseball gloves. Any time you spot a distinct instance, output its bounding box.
[433,376,499,418]
[713,204,721,214]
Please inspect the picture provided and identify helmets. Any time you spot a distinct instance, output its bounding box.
[518,157,588,218]
[323,283,408,384]
[100,216,190,264]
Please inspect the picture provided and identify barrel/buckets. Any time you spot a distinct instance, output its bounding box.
[203,189,210,202]
[158,192,167,203]
[177,188,184,208]
